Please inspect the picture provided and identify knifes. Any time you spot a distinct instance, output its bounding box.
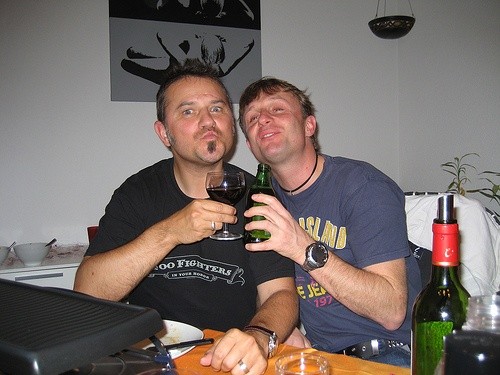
[146,338,214,352]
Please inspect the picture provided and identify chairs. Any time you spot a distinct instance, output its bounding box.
[405,193,488,297]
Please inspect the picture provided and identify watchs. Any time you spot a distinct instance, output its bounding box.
[299,241,328,273]
[241,324,279,358]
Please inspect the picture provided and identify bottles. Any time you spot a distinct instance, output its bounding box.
[410,218,472,375]
[249,163,275,244]
[444,295,500,375]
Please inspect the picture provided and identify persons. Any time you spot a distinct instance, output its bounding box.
[71,65,298,375]
[236,78,422,369]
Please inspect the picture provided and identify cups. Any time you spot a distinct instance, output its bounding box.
[87,226,98,243]
[275,353,329,375]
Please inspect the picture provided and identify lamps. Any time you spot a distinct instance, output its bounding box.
[368,0,416,39]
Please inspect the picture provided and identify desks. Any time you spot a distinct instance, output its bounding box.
[0,243,90,290]
[172,329,411,375]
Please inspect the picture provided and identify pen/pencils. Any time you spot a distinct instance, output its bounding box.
[145,337,214,352]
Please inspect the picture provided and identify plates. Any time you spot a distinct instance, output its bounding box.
[133,320,205,359]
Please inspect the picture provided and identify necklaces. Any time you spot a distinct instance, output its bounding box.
[278,151,318,196]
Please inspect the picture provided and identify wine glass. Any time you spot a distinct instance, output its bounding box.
[205,172,245,241]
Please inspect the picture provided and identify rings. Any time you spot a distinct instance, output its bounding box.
[237,360,249,374]
[211,222,215,231]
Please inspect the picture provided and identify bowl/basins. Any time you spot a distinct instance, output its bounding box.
[13,243,52,266]
[0,246,11,267]
